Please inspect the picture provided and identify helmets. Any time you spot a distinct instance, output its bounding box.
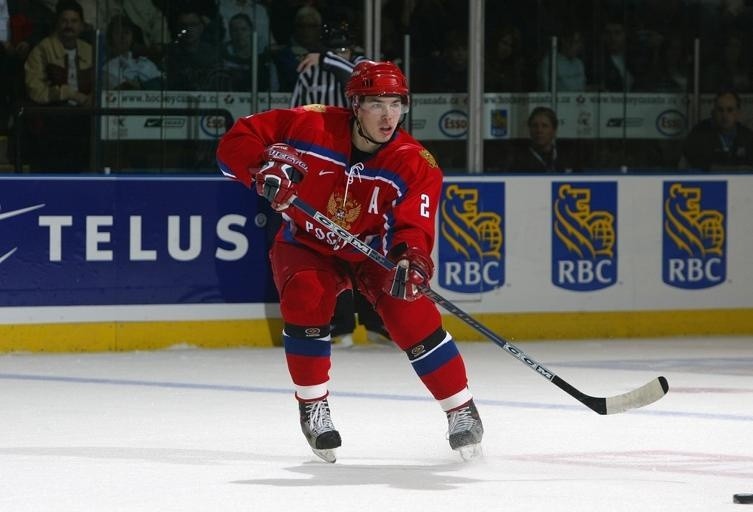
[319,22,354,52]
[344,59,409,99]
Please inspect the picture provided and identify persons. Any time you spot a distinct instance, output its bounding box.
[216,61,486,450]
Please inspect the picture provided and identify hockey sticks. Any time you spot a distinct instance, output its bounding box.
[286,194,668,414]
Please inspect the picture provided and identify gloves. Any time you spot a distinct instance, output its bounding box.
[255,142,309,210]
[380,241,435,303]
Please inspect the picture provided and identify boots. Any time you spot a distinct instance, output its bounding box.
[299,398,342,449]
[446,399,484,450]
